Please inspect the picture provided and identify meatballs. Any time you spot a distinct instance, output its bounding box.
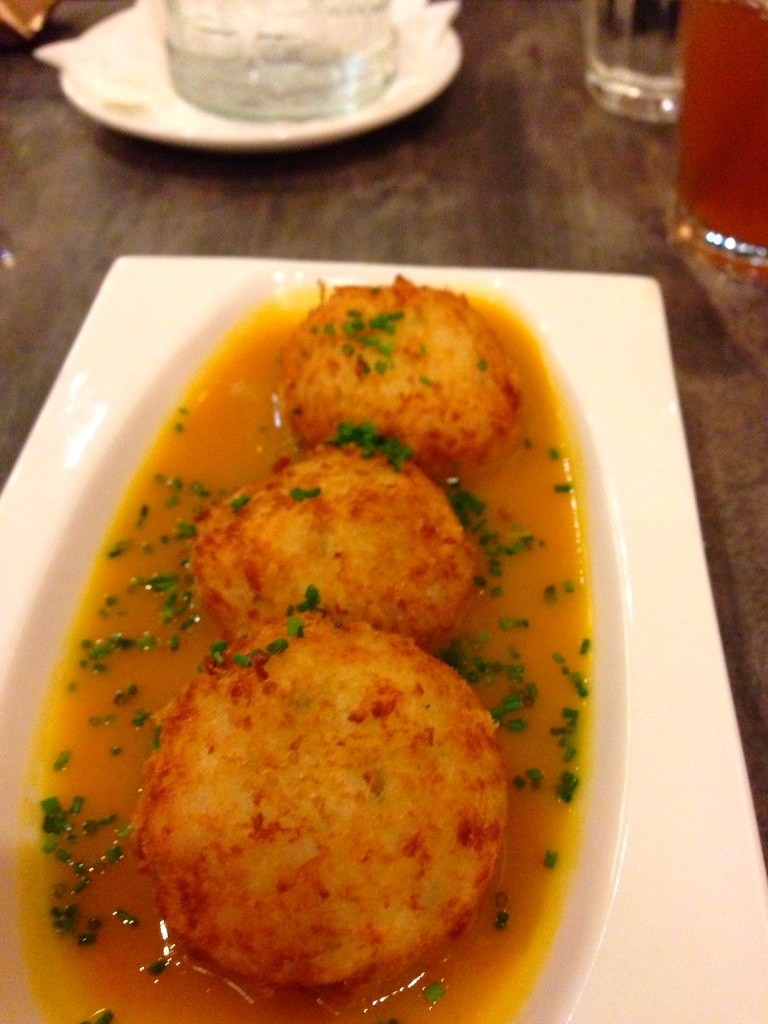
[129,607,509,986]
[278,274,525,479]
[191,443,480,665]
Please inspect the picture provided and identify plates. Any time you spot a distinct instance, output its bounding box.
[0,256,768,1024]
[61,0,461,150]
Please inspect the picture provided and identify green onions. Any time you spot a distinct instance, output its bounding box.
[36,305,592,1024]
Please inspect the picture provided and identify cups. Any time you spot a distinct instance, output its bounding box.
[164,0,399,121]
[580,0,681,123]
[670,0,768,275]
